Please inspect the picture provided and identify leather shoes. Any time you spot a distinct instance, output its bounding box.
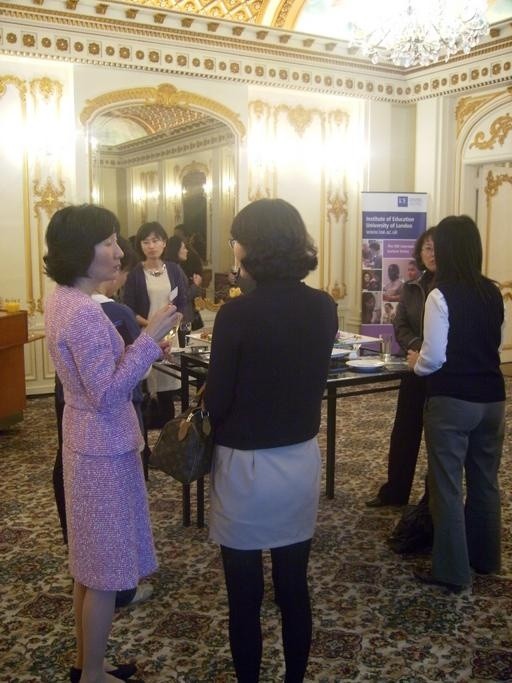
[412,566,473,593]
[71,663,137,682]
[365,492,390,507]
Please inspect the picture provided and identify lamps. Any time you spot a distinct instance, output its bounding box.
[323,0,489,67]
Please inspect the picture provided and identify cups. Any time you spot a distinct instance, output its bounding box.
[2,297,19,313]
[377,334,392,360]
[177,321,191,347]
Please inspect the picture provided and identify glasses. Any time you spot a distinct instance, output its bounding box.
[421,245,434,256]
[227,238,236,249]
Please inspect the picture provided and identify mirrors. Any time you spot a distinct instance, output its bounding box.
[86,98,241,305]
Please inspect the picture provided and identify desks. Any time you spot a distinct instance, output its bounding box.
[151,333,412,529]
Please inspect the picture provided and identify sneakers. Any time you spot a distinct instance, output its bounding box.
[129,584,154,606]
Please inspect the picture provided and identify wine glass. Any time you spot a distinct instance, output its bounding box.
[158,312,182,366]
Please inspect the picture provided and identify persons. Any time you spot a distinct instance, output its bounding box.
[114,221,201,429]
[41,206,184,682]
[367,225,441,508]
[361,242,423,325]
[408,215,506,591]
[53,234,156,612]
[205,194,339,683]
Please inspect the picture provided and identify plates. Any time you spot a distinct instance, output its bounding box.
[191,346,208,353]
[385,363,407,369]
[347,358,383,374]
[331,347,349,358]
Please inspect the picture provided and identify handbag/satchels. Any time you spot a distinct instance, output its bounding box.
[387,475,434,554]
[148,381,214,484]
